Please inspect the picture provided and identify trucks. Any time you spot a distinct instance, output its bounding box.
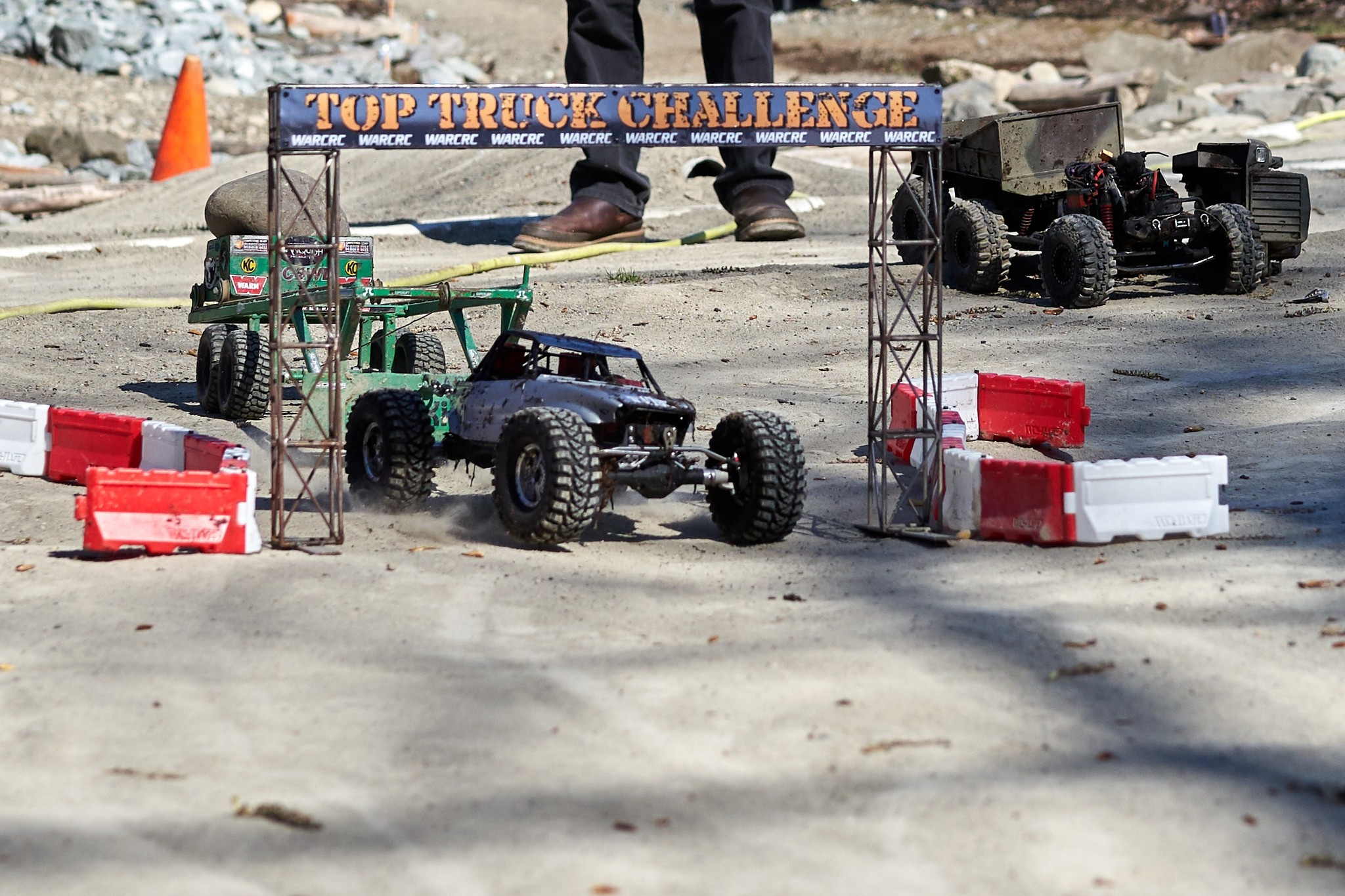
[885,100,1319,297]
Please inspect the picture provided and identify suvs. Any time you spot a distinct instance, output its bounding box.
[349,322,811,551]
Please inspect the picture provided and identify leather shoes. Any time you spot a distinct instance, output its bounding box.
[513,196,646,252]
[733,185,805,241]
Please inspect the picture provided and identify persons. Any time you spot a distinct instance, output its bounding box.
[513,0,806,253]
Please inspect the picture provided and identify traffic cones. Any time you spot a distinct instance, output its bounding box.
[150,54,214,184]
